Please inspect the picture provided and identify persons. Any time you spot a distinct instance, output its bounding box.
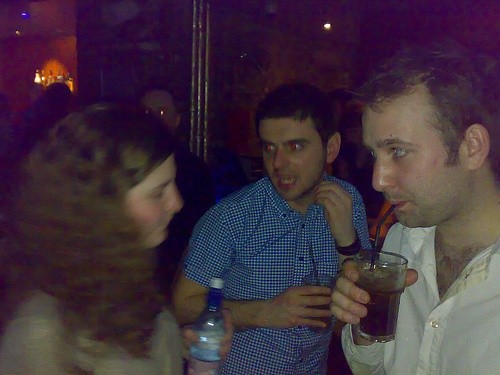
[169,82,374,375]
[0,77,215,375]
[329,42,500,375]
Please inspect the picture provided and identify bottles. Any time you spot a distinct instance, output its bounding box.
[34,69,73,92]
[187,277,224,375]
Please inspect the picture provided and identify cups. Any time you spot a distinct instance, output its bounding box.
[351,250,408,343]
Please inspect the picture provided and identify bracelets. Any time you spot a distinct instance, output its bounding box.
[335,229,362,256]
